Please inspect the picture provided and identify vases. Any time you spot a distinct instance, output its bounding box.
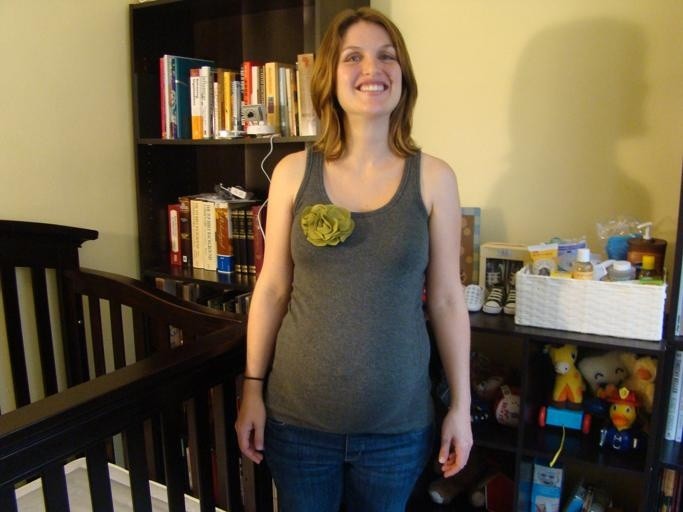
[301,203,355,247]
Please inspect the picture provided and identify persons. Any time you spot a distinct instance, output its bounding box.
[234,7,474,512]
[537,469,561,488]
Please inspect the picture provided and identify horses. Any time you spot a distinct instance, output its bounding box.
[544,403,585,431]
[528,458,565,512]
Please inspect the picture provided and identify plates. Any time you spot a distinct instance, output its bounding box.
[428,311,683,512]
[129,0,371,512]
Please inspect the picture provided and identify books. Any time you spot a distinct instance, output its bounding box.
[514,262,667,344]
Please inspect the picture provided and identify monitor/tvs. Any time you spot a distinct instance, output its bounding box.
[661,350,683,510]
[168,192,267,285]
[159,52,319,140]
[155,278,253,346]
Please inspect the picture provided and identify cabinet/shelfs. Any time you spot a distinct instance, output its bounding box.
[604,261,637,282]
[572,247,594,281]
[638,256,658,283]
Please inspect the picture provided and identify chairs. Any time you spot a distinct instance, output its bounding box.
[463,283,483,315]
[507,263,524,290]
[482,288,503,317]
[505,289,518,315]
[486,262,505,292]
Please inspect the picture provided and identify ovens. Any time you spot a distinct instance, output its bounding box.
[0,218,272,512]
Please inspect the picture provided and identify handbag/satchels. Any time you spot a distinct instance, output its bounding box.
[243,376,265,381]
